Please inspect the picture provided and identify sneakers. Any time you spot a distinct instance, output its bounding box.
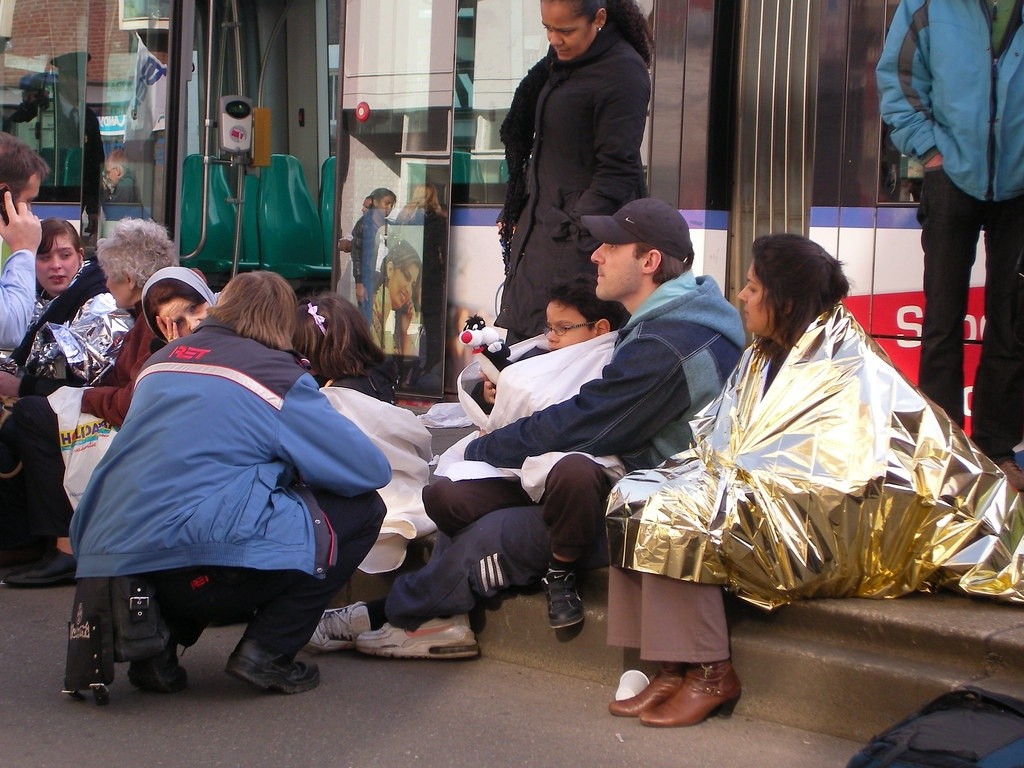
[539,568,585,629]
[355,611,480,659]
[299,601,371,654]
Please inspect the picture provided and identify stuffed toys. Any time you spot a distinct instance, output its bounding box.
[458,315,513,371]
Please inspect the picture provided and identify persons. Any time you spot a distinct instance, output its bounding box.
[0,219,177,588]
[51,51,105,194]
[308,198,745,657]
[604,233,867,728]
[103,148,136,203]
[350,183,446,358]
[0,216,110,412]
[493,0,651,346]
[69,271,398,694]
[875,0,1024,488]
[0,132,50,349]
[141,266,221,345]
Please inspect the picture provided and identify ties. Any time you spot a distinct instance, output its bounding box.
[71,107,80,130]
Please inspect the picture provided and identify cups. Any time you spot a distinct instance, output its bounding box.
[615,669,650,702]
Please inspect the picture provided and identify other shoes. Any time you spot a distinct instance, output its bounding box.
[3,545,78,585]
[0,542,45,566]
[1001,459,1024,491]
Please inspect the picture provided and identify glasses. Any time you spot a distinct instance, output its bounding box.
[543,320,597,336]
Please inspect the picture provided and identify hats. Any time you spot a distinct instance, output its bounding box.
[19,72,57,89]
[582,197,692,262]
[50,51,91,79]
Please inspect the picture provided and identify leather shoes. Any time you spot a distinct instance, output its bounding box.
[128,639,187,695]
[225,636,321,693]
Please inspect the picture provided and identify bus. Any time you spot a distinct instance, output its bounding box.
[1,0,1023,439]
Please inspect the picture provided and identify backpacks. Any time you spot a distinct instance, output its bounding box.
[846,687,1024,768]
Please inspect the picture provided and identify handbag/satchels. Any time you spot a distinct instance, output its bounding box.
[61,576,170,706]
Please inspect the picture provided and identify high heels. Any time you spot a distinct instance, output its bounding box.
[608,661,690,717]
[637,659,741,727]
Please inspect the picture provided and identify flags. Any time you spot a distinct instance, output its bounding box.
[122,41,168,141]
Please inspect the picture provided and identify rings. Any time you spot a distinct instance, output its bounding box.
[169,336,173,339]
